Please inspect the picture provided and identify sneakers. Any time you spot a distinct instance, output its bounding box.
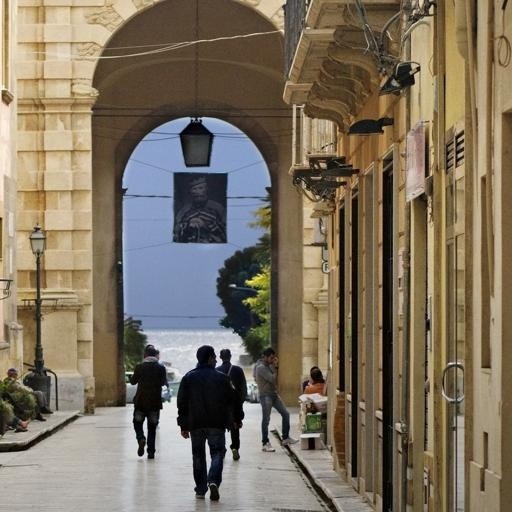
[41,408,53,414]
[35,414,46,421]
[262,442,275,452]
[281,434,299,446]
[232,449,239,460]
[138,438,146,456]
[195,482,219,500]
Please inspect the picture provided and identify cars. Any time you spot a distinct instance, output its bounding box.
[123,371,172,402]
[246,383,259,405]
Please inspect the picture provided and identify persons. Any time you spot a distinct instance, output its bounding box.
[215,349,247,460]
[304,369,326,394]
[302,366,318,392]
[128,344,166,459]
[173,177,226,242]
[176,345,235,501]
[0,368,53,433]
[253,348,299,453]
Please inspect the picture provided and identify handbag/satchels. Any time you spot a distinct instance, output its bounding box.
[161,384,171,402]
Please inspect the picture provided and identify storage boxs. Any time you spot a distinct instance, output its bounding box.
[300,433,327,450]
[305,415,322,431]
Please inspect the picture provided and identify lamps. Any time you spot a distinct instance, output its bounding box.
[346,117,394,134]
[377,63,420,97]
[179,0,214,167]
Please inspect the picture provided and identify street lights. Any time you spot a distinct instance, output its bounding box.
[23,222,51,410]
[228,284,258,294]
